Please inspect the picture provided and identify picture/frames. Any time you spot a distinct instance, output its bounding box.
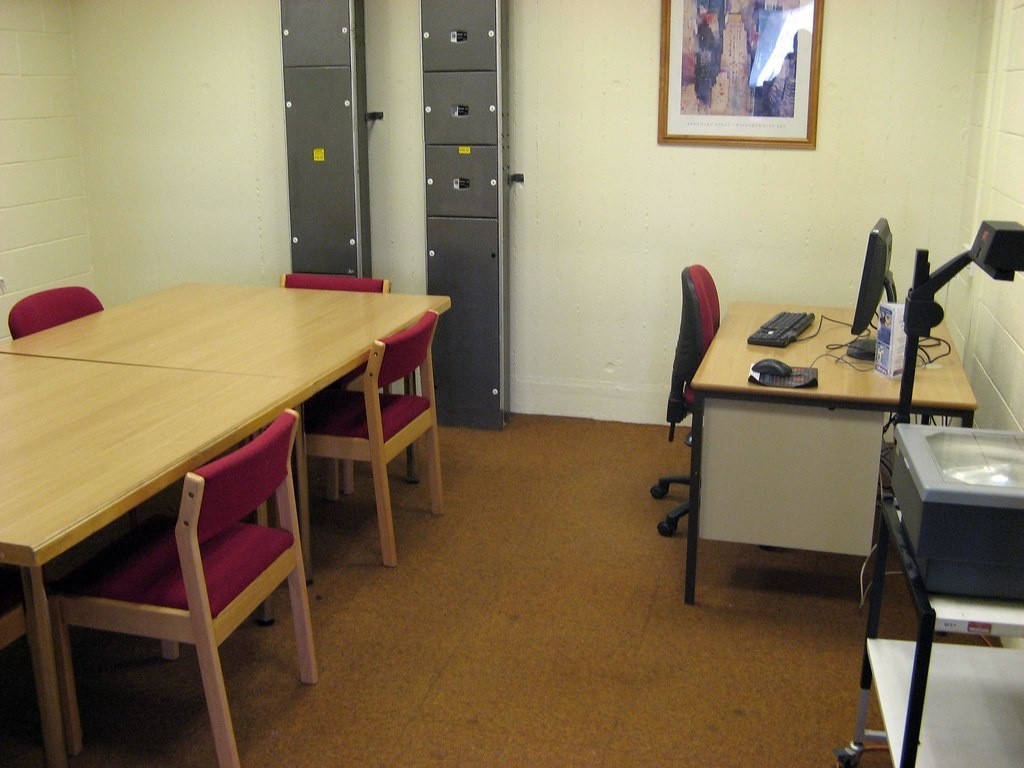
[657,0,823,150]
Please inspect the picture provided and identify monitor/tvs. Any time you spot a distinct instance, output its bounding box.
[845,217,898,361]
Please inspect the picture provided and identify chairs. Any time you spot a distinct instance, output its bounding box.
[650,264,721,537]
[8,286,104,340]
[49,407,319,768]
[253,272,445,626]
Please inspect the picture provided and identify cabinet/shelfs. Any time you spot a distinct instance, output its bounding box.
[833,494,1023,768]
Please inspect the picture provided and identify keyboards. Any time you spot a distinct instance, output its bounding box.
[747,311,815,348]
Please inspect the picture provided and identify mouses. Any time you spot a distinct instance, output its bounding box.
[752,359,792,377]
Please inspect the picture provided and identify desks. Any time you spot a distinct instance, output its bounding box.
[684,301,977,606]
[0,282,452,768]
